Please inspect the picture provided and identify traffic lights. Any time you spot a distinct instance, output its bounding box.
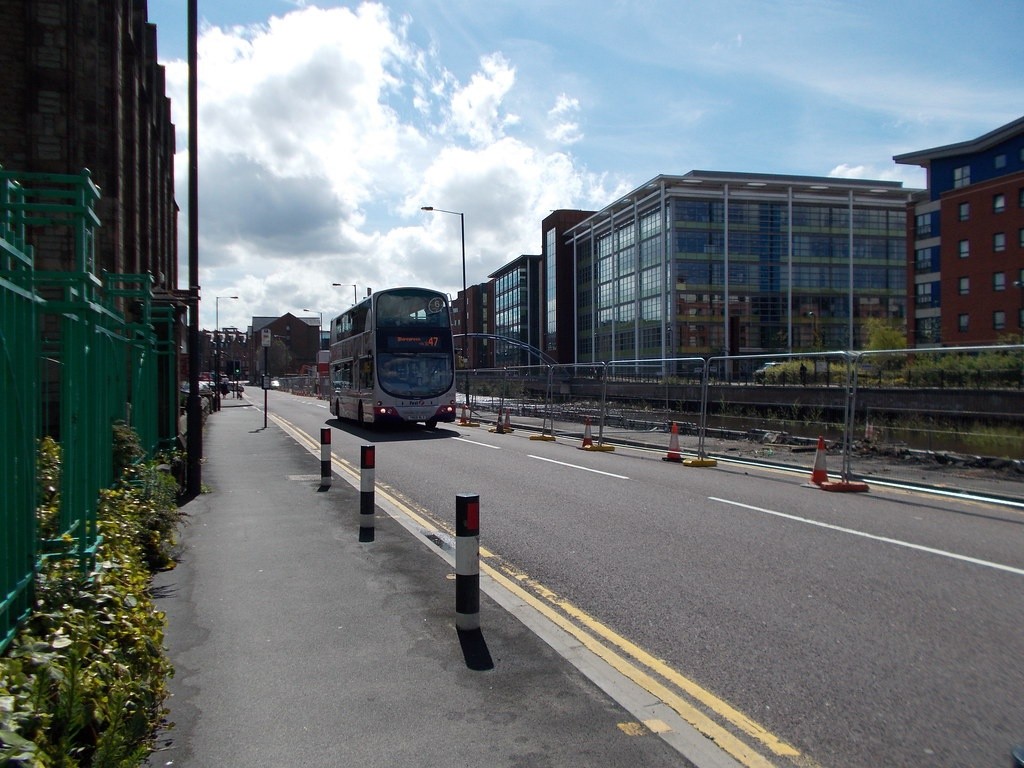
[234,360,241,375]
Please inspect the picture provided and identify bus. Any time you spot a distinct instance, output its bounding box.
[329,287,457,432]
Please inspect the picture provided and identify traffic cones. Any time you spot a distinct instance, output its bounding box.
[493,409,506,434]
[504,409,515,430]
[577,418,593,451]
[460,404,467,424]
[800,436,829,489]
[661,422,685,462]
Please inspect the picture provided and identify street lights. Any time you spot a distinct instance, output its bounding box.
[216,297,238,411]
[303,309,322,349]
[421,206,469,406]
